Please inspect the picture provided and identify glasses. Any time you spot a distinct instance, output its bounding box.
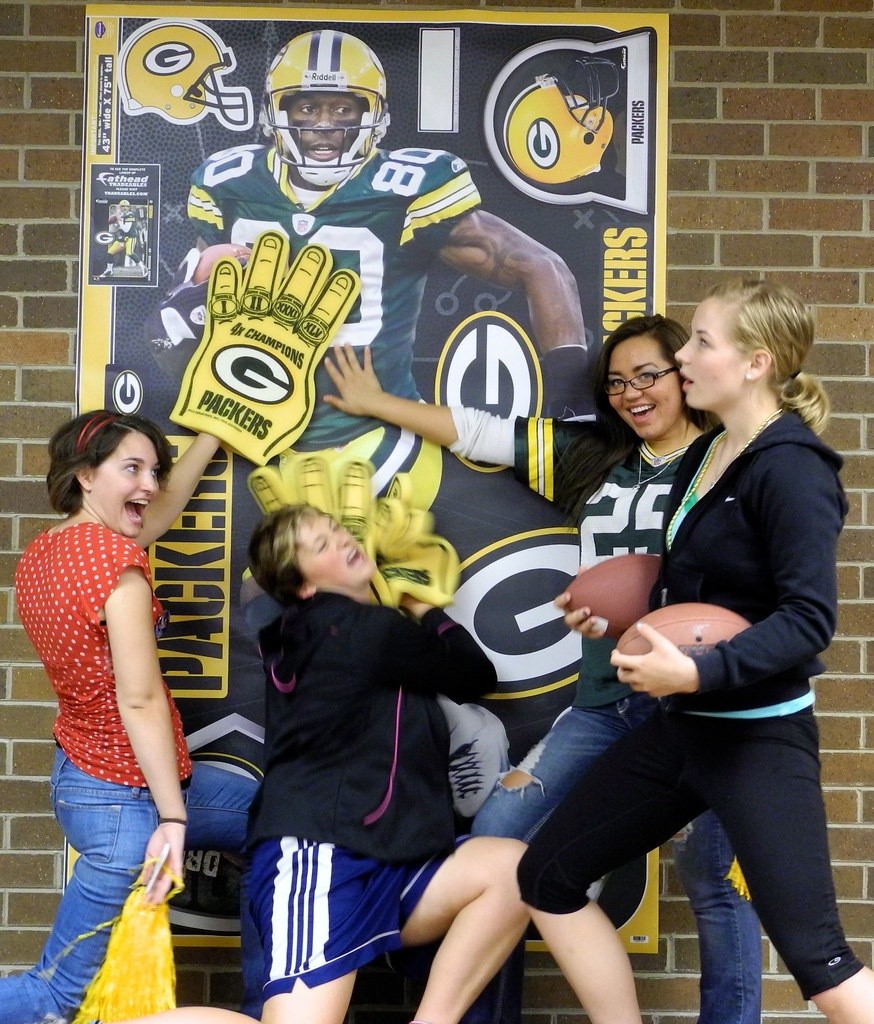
[604,366,679,395]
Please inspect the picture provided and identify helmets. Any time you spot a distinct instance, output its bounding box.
[119,199,131,214]
[260,28,387,186]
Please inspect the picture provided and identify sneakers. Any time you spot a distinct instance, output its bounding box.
[101,270,114,277]
[141,266,149,277]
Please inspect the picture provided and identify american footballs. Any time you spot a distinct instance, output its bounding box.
[562,554,662,640]
[192,243,252,286]
[614,601,755,659]
[107,214,117,224]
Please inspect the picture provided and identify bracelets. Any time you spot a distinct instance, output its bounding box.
[159,818,189,826]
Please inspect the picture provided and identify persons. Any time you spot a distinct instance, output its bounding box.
[125,503,530,1024]
[0,410,261,1024]
[323,314,764,1024]
[98,199,148,279]
[515,280,874,1024]
[140,29,596,642]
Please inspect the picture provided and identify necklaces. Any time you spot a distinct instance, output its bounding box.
[632,444,684,491]
[667,410,782,551]
